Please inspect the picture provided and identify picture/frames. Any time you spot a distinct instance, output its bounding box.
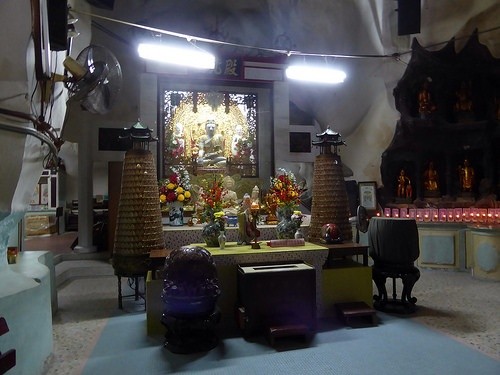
[358,182,376,211]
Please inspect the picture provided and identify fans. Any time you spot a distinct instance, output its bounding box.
[348,205,369,244]
[43,45,124,115]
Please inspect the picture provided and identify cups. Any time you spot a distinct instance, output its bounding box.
[7,246,18,264]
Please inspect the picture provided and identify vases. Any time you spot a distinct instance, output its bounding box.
[226,215,239,227]
[168,208,183,227]
[227,157,254,176]
[166,157,183,176]
[202,220,226,248]
[160,308,219,355]
[276,210,303,239]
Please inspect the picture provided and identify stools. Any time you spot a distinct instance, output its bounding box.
[371,265,420,314]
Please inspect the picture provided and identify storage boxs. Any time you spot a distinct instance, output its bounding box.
[236,259,317,338]
[383,207,500,224]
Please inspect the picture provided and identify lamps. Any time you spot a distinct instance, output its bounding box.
[285,56,347,85]
[137,32,216,71]
[397,170,413,200]
[455,81,473,112]
[423,163,440,193]
[460,160,475,192]
[418,90,434,121]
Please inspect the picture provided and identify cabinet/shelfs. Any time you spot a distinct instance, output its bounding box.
[22,168,60,239]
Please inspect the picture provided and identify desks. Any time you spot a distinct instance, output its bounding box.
[146,236,372,343]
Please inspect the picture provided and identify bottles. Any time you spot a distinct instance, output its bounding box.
[218,231,226,249]
[242,193,251,209]
[252,185,259,201]
[295,228,304,239]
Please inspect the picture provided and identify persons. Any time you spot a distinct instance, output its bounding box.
[219,175,243,208]
[396,169,409,198]
[236,193,261,245]
[423,161,440,192]
[458,158,475,192]
[196,116,228,167]
[201,179,208,192]
[250,185,261,209]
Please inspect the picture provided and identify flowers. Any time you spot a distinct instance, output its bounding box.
[167,139,183,157]
[158,162,194,208]
[266,169,309,214]
[194,176,243,222]
[231,141,252,164]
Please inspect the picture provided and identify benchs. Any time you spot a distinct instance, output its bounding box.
[64,198,108,232]
